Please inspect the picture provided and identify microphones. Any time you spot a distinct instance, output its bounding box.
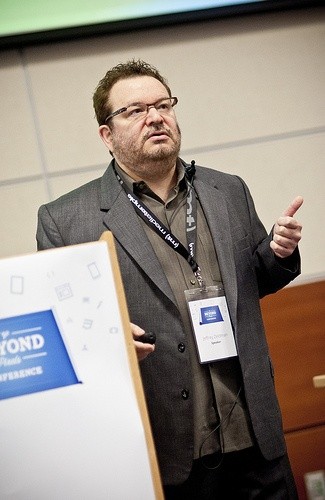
[190,160,196,176]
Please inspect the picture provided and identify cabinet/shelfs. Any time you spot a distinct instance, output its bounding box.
[259,272,325,499]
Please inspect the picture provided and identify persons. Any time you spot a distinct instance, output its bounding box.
[36,61,304,500]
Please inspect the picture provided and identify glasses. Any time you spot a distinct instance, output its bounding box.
[103,97,179,123]
